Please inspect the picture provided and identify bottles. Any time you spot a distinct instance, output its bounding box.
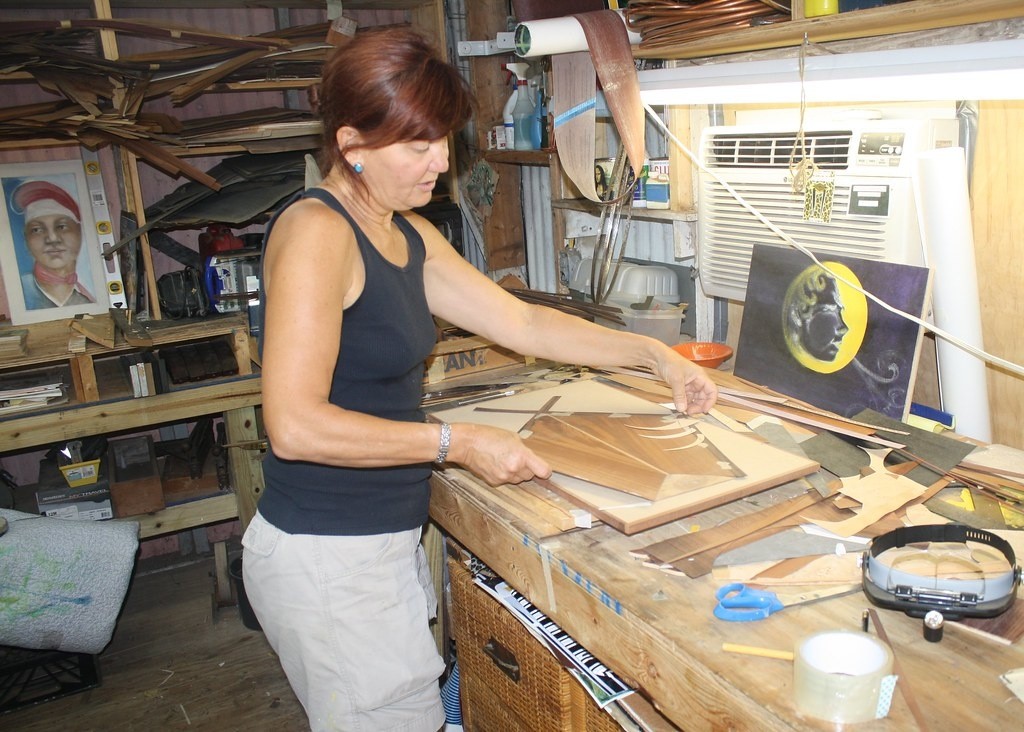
[629,158,647,207]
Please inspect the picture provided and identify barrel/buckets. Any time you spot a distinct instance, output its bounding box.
[595,157,616,201]
[228,556,262,629]
[645,171,670,209]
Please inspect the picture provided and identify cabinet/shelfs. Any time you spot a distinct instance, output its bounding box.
[0,0,694,616]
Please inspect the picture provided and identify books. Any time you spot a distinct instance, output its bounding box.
[0,328,70,415]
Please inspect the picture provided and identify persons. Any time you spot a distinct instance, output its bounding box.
[241,27,717,731]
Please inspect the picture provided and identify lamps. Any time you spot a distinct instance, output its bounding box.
[636,40,1024,107]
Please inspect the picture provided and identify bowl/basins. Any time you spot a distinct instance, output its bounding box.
[670,341,733,370]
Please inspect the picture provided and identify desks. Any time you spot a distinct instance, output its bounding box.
[418,367,1024,732]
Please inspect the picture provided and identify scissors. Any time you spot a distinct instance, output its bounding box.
[712,582,863,622]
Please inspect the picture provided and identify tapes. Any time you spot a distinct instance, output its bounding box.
[792,629,893,722]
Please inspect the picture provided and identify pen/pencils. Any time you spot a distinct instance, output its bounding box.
[720,643,795,662]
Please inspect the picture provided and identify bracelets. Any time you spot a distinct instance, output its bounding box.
[436,423,451,464]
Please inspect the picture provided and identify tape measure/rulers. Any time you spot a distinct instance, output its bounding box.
[670,408,746,478]
[516,396,562,433]
[472,406,674,418]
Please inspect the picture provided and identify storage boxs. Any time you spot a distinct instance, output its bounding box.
[108,435,166,518]
[593,296,685,345]
[426,336,526,382]
[36,459,113,522]
[155,446,226,501]
[57,458,101,488]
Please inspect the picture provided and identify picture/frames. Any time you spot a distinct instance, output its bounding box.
[0,160,111,325]
